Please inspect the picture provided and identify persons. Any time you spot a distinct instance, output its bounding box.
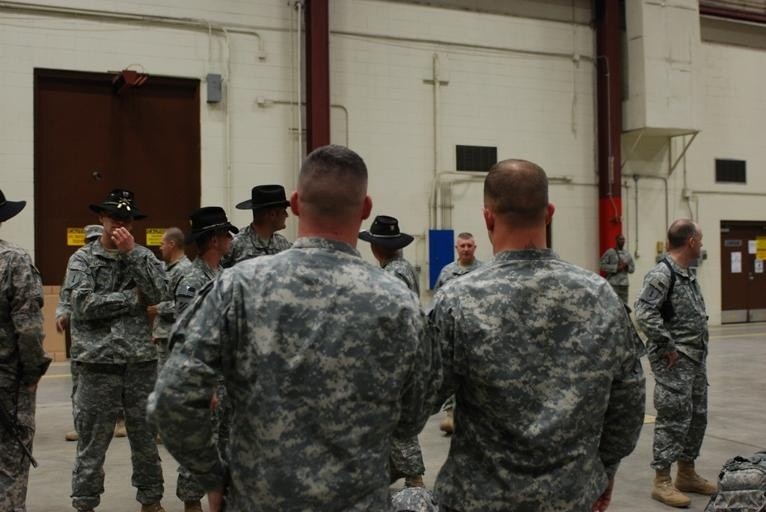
[149,144,432,512]
[0,189,53,512]
[433,231,487,435]
[633,218,718,508]
[59,184,290,511]
[389,157,647,512]
[599,233,636,306]
[357,216,427,487]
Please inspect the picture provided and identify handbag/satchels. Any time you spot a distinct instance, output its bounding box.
[705,452,766,512]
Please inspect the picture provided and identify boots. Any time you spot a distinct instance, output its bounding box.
[651,464,690,507]
[440,409,455,434]
[186,502,202,511]
[675,460,716,493]
[140,501,164,511]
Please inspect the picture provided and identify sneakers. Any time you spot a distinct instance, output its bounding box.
[66,432,79,439]
[114,424,129,437]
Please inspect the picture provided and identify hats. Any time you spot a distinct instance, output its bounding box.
[89,189,146,219]
[1,190,25,222]
[83,225,104,239]
[184,206,239,240]
[358,216,414,248]
[236,184,291,209]
